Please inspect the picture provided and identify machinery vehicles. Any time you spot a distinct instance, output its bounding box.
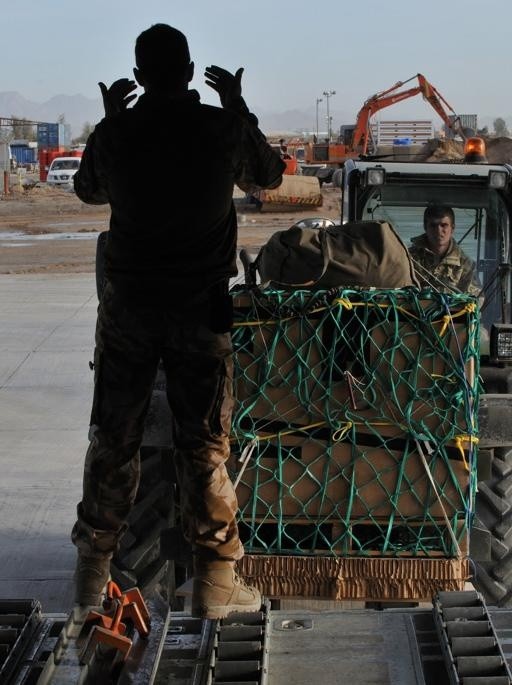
[298,72,476,181]
[246,134,323,212]
[211,156,510,606]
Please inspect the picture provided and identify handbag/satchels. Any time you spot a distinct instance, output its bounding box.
[255,218,422,290]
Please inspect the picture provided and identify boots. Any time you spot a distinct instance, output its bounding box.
[192,561,262,619]
[72,552,112,606]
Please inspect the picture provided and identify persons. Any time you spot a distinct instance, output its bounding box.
[407,204,485,309]
[69,22,287,622]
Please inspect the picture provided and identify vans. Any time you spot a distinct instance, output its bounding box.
[46,156,81,191]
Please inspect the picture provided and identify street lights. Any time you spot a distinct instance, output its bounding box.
[314,89,335,144]
